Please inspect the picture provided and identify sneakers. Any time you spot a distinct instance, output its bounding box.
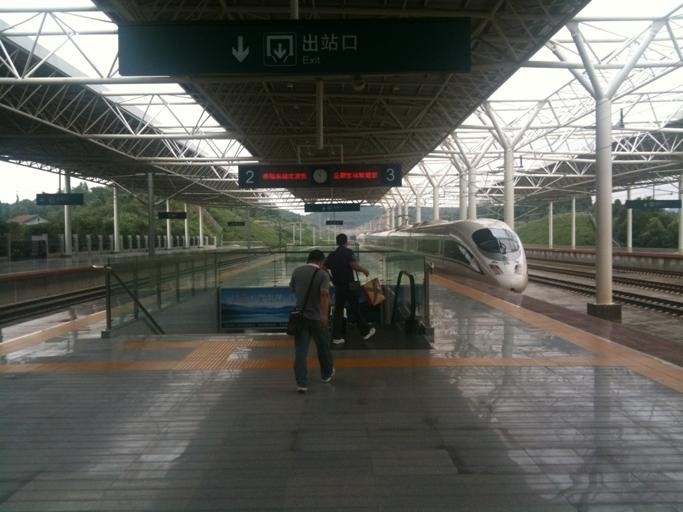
[363,328,376,339]
[333,339,345,344]
[321,368,335,382]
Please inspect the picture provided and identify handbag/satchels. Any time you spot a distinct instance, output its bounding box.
[287,310,303,335]
[349,278,386,308]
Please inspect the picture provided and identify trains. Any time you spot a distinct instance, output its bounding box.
[356,216,529,293]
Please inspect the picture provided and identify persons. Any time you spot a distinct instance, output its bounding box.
[322,233,376,345]
[286,248,336,393]
